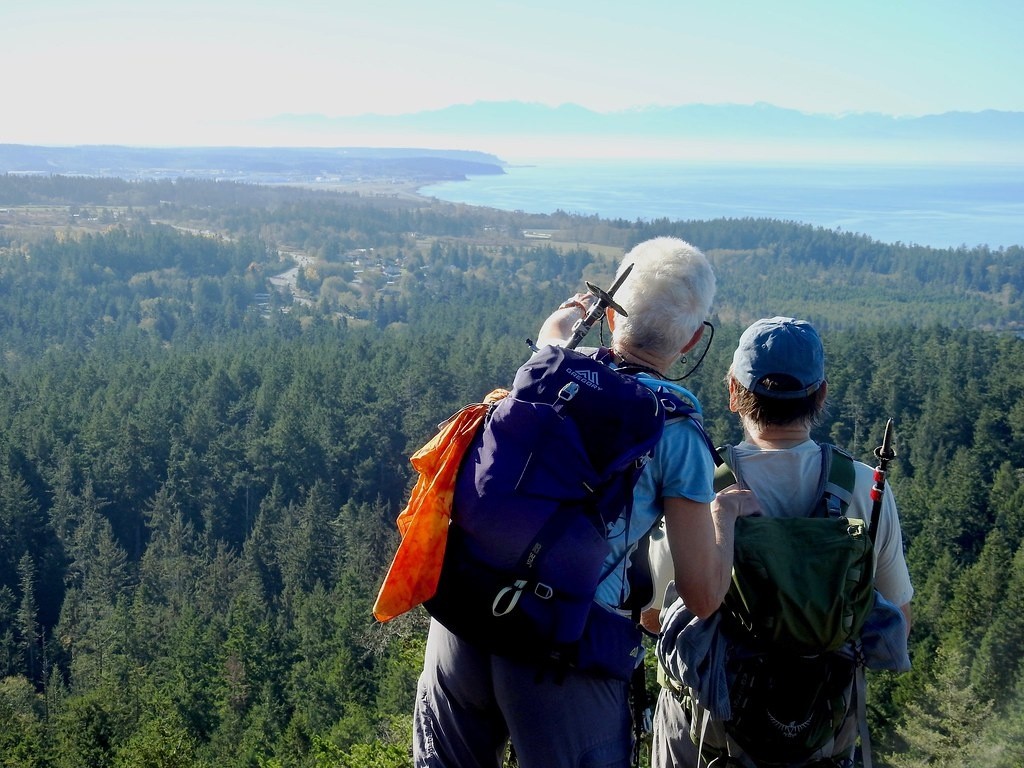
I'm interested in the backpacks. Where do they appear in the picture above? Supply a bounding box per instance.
[421,344,724,685]
[715,443,878,768]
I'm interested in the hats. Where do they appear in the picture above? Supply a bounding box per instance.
[733,316,824,399]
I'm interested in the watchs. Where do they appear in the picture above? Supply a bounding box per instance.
[558,299,586,317]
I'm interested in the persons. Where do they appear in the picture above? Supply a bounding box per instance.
[413,235,752,768]
[640,315,914,768]
